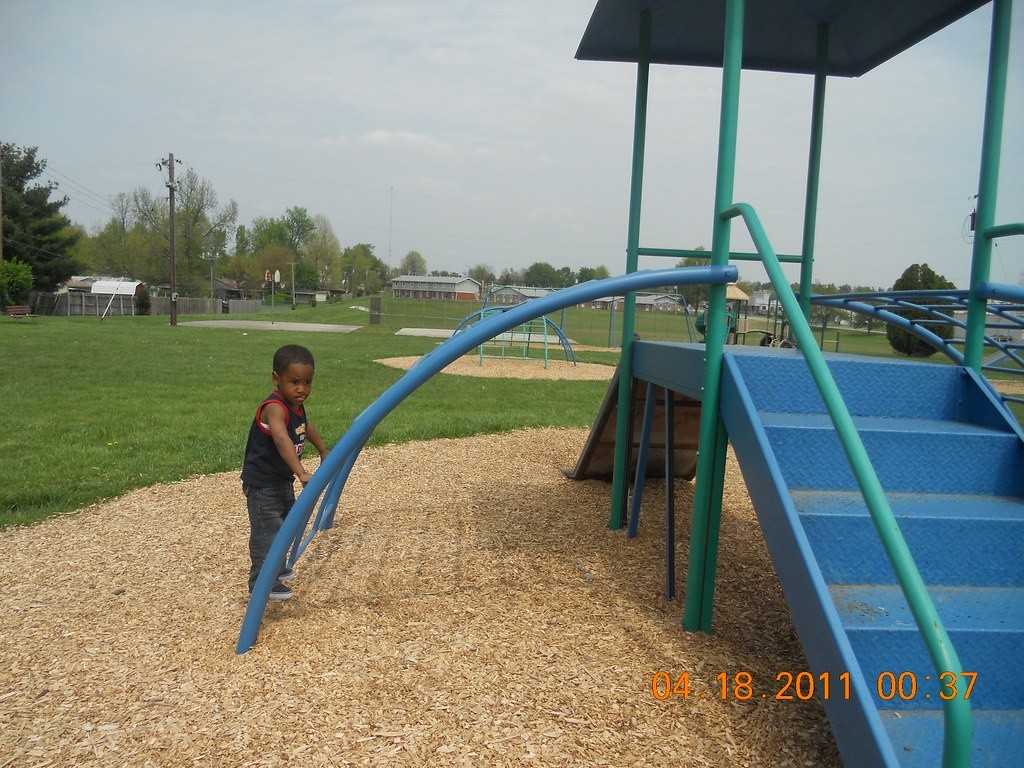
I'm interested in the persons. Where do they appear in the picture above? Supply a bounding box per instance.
[240,344,330,598]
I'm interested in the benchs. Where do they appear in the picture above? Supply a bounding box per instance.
[7,305,38,323]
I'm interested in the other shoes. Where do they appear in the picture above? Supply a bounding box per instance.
[250,578,293,599]
[278,569,295,581]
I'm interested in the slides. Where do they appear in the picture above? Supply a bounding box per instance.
[560,332,702,482]
